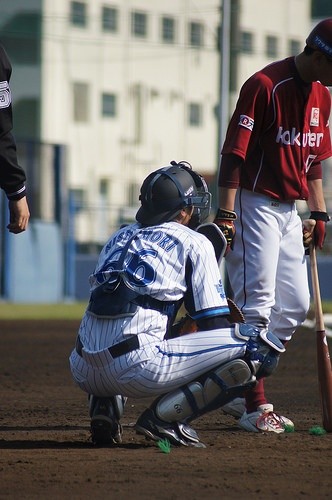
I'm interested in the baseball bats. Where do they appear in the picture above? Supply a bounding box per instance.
[303,217,332,433]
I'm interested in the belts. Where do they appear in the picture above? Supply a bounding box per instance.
[73,336,139,362]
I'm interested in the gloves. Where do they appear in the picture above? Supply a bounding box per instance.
[302,210,332,255]
[212,207,237,257]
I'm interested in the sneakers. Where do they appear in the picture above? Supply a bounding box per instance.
[87,397,126,449]
[238,403,285,435]
[133,407,191,448]
[221,396,246,419]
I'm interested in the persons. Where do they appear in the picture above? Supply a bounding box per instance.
[214,18,331,434]
[0,45,30,235]
[68,159,286,446]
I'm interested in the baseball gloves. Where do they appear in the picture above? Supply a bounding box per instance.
[180,296,246,338]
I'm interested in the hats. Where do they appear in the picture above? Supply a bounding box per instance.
[135,164,199,226]
[306,17,332,55]
